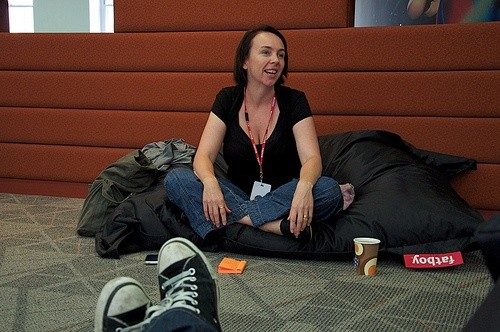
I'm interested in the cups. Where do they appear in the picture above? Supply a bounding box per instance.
[353,238,381,276]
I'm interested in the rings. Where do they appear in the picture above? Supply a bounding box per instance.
[303,214,311,218]
[219,206,226,209]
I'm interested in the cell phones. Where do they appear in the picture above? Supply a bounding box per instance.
[144,254,158,264]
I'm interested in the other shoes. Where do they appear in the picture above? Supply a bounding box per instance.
[280,217,313,242]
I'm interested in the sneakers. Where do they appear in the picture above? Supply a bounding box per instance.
[156,237,223,332]
[94,276,161,332]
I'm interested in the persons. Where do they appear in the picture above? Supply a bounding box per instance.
[93,236,224,332]
[162,24,344,243]
